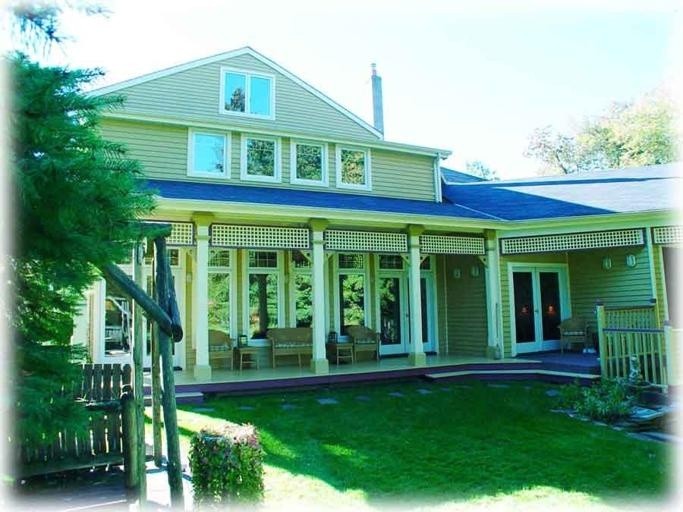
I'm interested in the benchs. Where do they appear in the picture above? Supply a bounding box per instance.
[263,328,313,369]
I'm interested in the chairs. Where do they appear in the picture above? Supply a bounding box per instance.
[344,324,380,363]
[206,330,233,372]
[556,316,592,355]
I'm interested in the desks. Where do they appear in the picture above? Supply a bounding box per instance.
[325,342,353,368]
[233,346,259,372]
